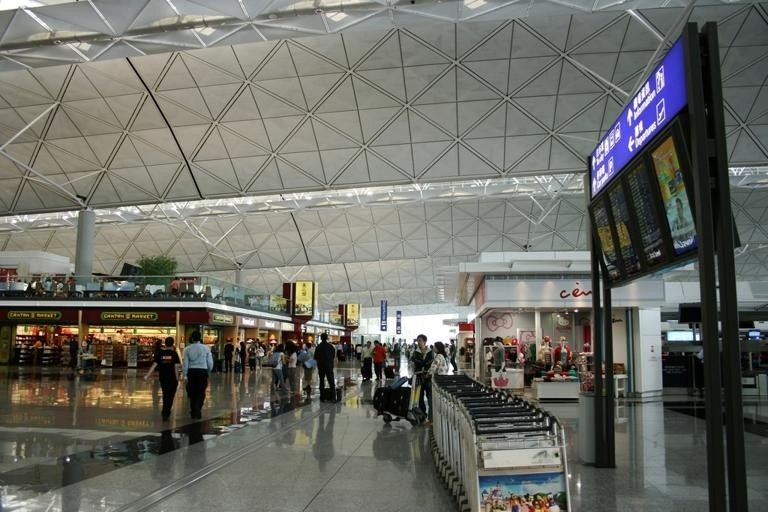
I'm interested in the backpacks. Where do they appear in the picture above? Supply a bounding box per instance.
[268,352,279,367]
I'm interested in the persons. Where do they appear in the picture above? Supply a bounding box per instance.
[32,335,43,364]
[148,374,162,414]
[9,272,75,299]
[553,339,571,365]
[425,340,449,376]
[40,336,48,347]
[200,336,458,392]
[478,478,562,512]
[143,336,184,422]
[670,197,693,243]
[153,339,165,373]
[488,336,507,372]
[409,334,437,426]
[68,335,78,368]
[539,338,556,364]
[268,394,316,449]
[181,417,209,472]
[169,276,194,299]
[182,330,214,420]
[140,422,179,489]
[311,332,337,404]
[310,400,339,472]
[209,367,411,421]
[407,420,432,507]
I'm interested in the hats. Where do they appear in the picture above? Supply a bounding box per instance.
[492,336,503,342]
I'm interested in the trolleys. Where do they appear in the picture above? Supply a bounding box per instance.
[377,367,428,426]
[428,368,577,512]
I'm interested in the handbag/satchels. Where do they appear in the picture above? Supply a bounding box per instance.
[447,360,454,376]
[494,378,509,386]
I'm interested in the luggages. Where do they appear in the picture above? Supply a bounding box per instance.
[324,389,342,401]
[384,360,395,378]
[373,387,410,416]
[360,361,372,378]
